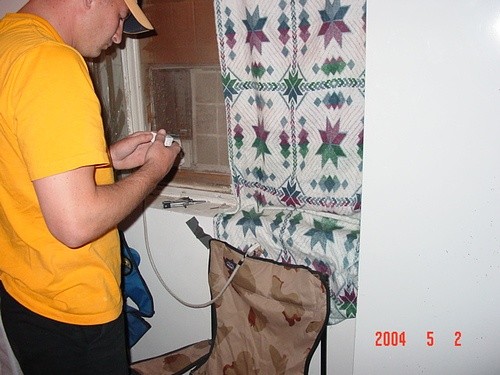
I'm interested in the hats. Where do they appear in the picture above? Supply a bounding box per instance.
[124,0,155,36]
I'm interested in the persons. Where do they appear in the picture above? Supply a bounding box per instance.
[0,0,181,375]
